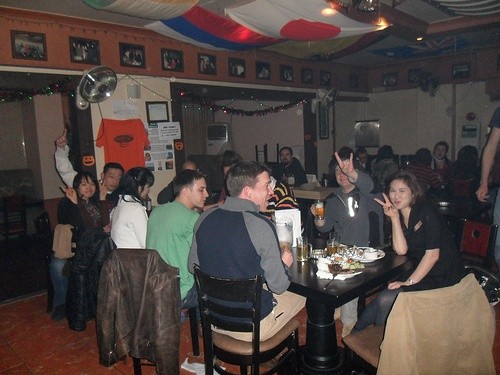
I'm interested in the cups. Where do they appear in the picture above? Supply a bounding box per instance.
[296,236,313,262]
[329,232,340,243]
[326,240,340,256]
[314,199,324,220]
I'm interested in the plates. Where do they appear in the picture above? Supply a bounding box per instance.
[346,247,387,262]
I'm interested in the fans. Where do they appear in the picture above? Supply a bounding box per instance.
[77,66,118,110]
[417,71,438,96]
[318,87,338,109]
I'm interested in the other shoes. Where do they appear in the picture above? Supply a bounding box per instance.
[342,319,357,337]
[333,306,340,321]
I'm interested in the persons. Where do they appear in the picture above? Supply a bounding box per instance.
[475,107,500,275]
[46,127,496,336]
[187,162,341,343]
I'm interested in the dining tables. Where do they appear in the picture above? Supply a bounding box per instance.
[290,186,337,212]
[281,248,416,375]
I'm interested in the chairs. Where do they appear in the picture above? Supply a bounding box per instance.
[0,196,26,249]
[36,153,500,375]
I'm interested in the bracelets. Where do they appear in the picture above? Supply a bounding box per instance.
[406,278,414,286]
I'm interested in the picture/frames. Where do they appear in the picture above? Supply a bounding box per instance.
[256,62,270,80]
[197,53,216,74]
[228,57,246,77]
[302,68,313,83]
[11,30,48,61]
[280,65,293,81]
[69,37,101,65]
[119,43,145,68]
[161,48,184,72]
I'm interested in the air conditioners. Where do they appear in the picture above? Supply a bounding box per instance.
[206,124,229,155]
